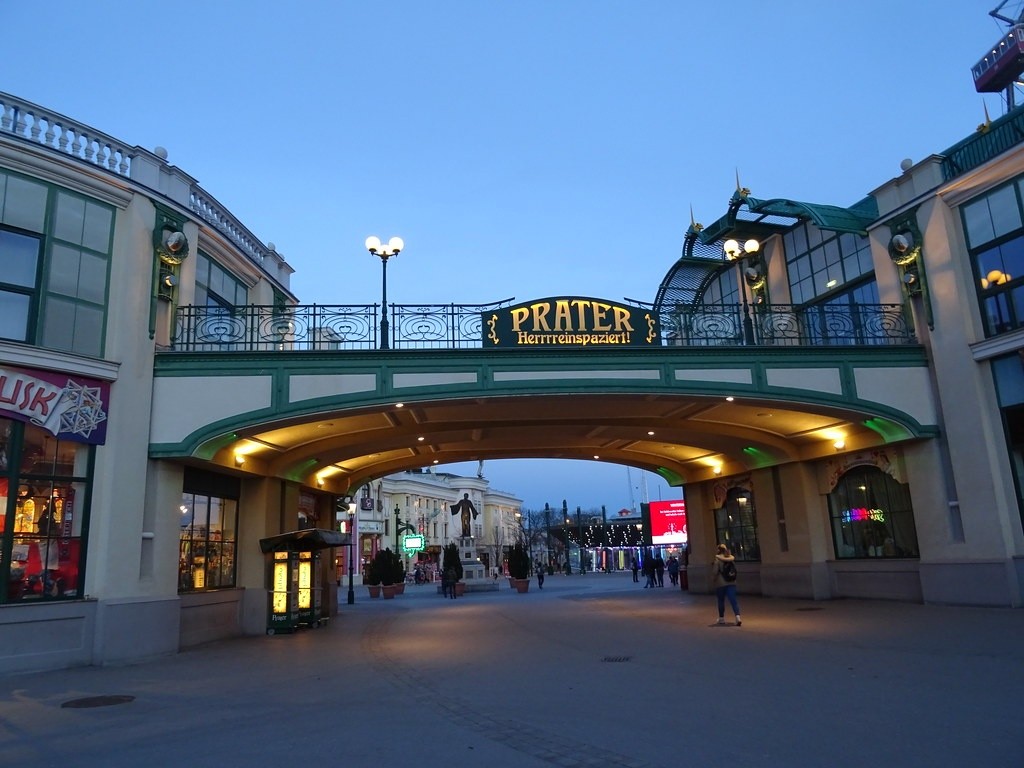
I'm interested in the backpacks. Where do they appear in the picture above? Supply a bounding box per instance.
[718,562,737,581]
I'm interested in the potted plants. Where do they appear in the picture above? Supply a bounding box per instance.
[515,544,530,593]
[375,547,396,599]
[509,545,515,589]
[394,554,407,594]
[445,542,466,597]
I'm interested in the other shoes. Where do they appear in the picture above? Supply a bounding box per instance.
[717,619,725,624]
[734,617,742,626]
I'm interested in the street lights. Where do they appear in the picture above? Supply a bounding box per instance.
[365,235,405,349]
[345,501,356,606]
[724,239,760,343]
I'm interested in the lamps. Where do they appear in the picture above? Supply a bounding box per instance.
[903,274,916,284]
[235,456,245,467]
[317,479,325,488]
[368,560,381,598]
[753,295,764,305]
[166,275,177,286]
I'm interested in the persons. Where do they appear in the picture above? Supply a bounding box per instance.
[413,563,422,584]
[629,553,679,587]
[537,562,572,589]
[447,569,458,599]
[712,544,742,626]
